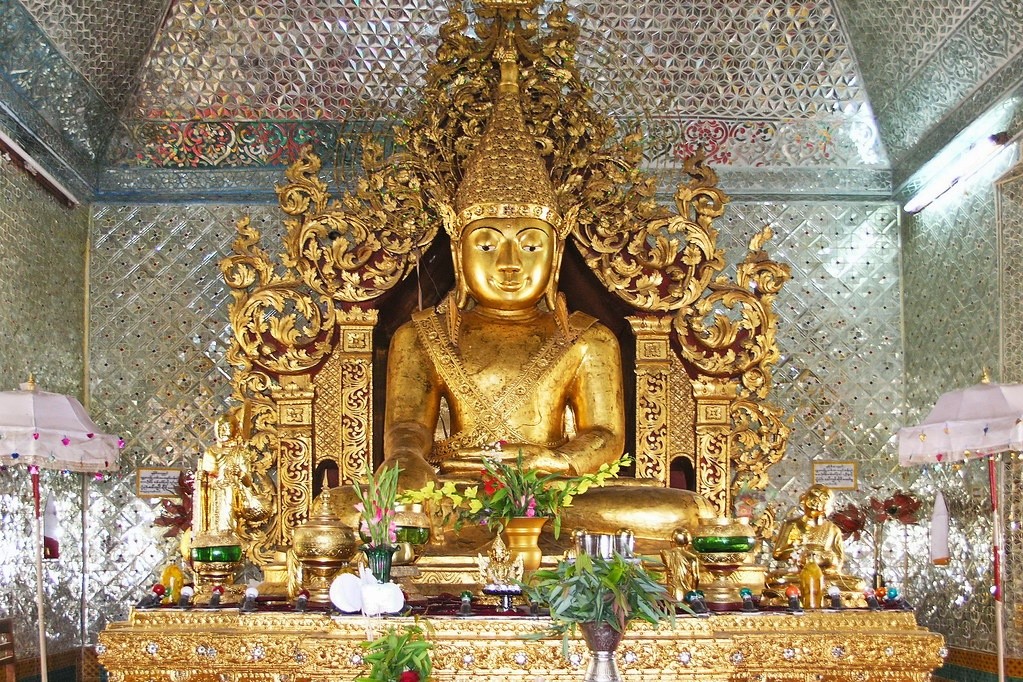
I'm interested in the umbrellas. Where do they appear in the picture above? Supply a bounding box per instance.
[0,373,121,682]
[894,363,1022,682]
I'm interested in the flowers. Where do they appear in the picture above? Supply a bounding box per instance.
[392,440,635,541]
[348,459,406,569]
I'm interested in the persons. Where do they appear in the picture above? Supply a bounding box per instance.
[189,410,264,542]
[770,486,861,594]
[313,48,719,591]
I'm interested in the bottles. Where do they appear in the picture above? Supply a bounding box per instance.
[798,553,823,609]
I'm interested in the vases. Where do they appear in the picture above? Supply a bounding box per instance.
[358,542,402,584]
[494,515,551,574]
